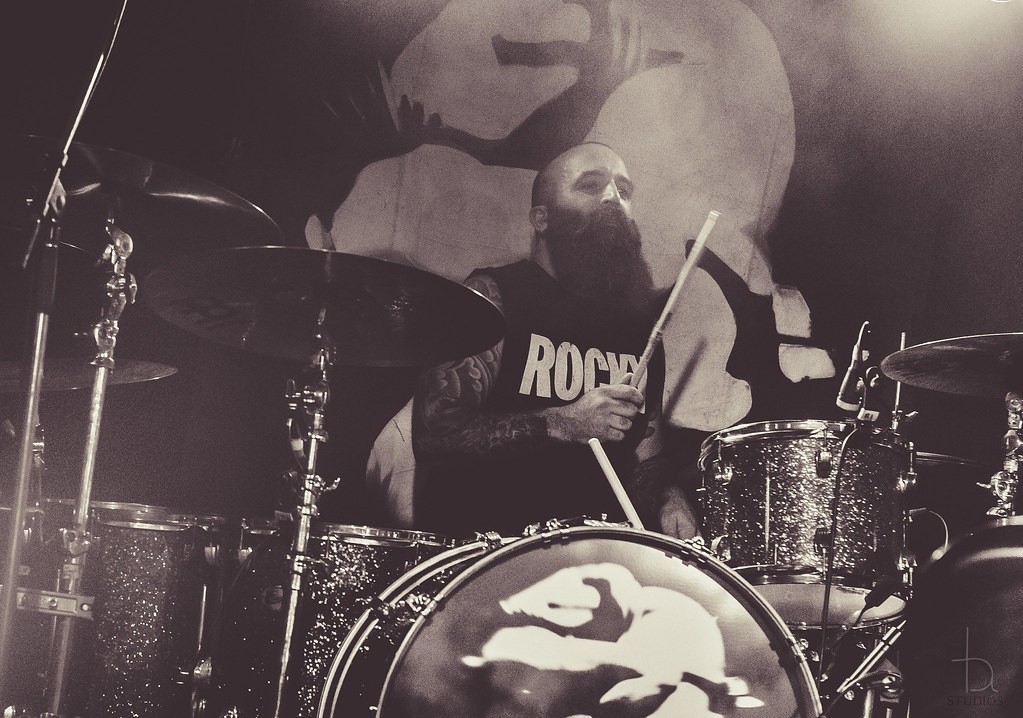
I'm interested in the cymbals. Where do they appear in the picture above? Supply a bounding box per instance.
[1,177,280,394]
[145,243,508,367]
[879,333,1022,395]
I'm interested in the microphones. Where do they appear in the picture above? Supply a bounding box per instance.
[836,321,872,412]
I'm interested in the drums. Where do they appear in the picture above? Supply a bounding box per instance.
[749,583,905,716]
[12,494,259,717]
[229,514,444,717]
[694,417,920,629]
[313,510,824,718]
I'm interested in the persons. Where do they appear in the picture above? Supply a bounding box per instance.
[412,142,704,548]
